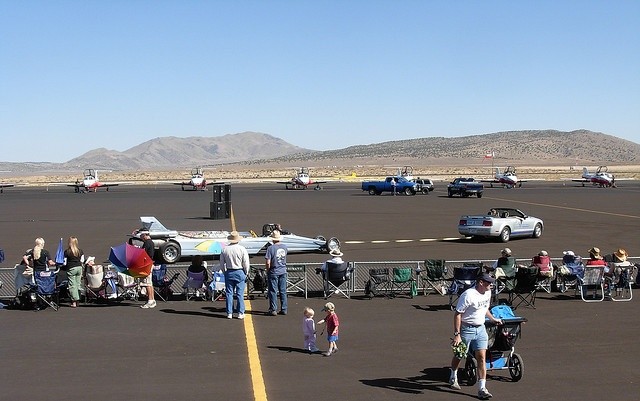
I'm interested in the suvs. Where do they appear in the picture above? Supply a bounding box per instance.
[408,179,434,194]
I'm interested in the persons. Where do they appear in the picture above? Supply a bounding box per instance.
[605,248,631,296]
[22,237,57,310]
[529,251,553,267]
[570,247,605,296]
[64,237,85,308]
[556,250,576,275]
[321,248,345,296]
[492,257,516,290]
[186,256,209,284]
[264,230,289,316]
[317,302,339,357]
[303,308,319,353]
[484,248,514,271]
[220,231,251,319]
[139,232,157,309]
[84,255,95,267]
[448,271,504,399]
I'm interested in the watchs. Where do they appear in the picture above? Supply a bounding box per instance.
[454,332,460,335]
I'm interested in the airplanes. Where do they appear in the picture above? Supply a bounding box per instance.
[66,169,118,193]
[571,166,634,188]
[277,167,326,190]
[481,166,527,188]
[172,168,224,190]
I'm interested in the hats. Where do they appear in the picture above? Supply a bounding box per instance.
[501,248,511,257]
[136,230,149,236]
[83,256,95,264]
[267,230,284,241]
[321,302,334,311]
[563,250,577,257]
[614,248,628,262]
[329,249,343,256]
[538,250,548,256]
[227,231,243,243]
[588,246,603,259]
[474,274,496,283]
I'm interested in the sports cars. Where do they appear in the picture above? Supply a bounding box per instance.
[458,207,544,243]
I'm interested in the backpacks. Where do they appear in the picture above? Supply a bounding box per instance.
[619,265,629,289]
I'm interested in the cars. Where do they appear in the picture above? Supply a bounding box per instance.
[126,217,340,264]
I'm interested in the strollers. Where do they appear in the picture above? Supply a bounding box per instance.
[464,305,528,386]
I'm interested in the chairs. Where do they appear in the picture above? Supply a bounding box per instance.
[363,267,392,300]
[286,264,308,300]
[210,271,227,303]
[181,270,209,302]
[153,272,180,303]
[13,263,35,296]
[574,265,606,303]
[57,264,69,307]
[498,256,516,267]
[84,263,107,304]
[492,267,516,306]
[529,258,553,294]
[415,258,448,297]
[247,265,269,299]
[391,268,417,299]
[509,265,540,310]
[463,262,483,271]
[321,260,352,300]
[555,264,584,294]
[604,264,637,302]
[115,272,140,303]
[447,267,481,311]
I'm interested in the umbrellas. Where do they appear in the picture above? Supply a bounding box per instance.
[56,238,65,264]
[107,242,153,278]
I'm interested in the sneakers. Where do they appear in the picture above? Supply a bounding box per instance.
[279,310,288,315]
[478,388,492,399]
[325,351,332,356]
[140,301,157,308]
[332,349,339,354]
[228,313,233,318]
[449,377,461,389]
[268,310,277,316]
[238,312,244,319]
[108,294,117,299]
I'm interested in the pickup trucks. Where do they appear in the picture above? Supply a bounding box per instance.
[447,178,484,198]
[362,176,420,196]
